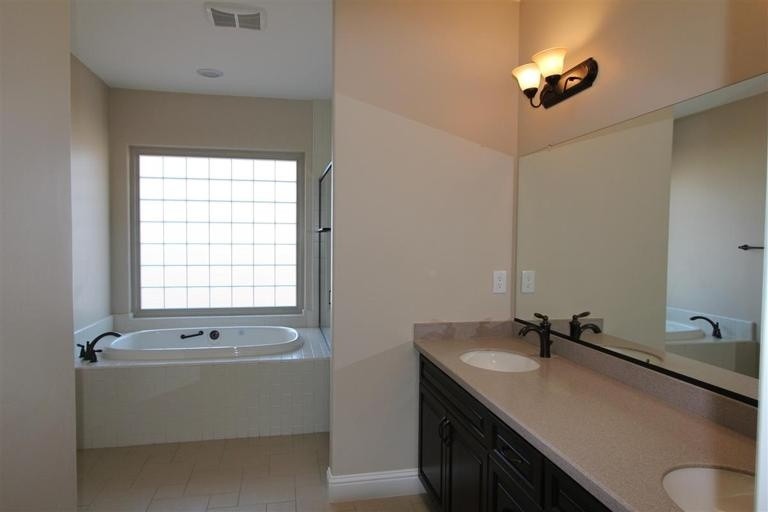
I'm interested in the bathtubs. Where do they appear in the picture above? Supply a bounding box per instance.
[667,320,705,340]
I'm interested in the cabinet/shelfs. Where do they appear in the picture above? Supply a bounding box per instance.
[417,354,489,512]
[491,415,612,512]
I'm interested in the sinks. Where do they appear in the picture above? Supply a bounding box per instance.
[459,348,541,374]
[103,325,305,360]
[604,345,662,363]
[663,463,756,512]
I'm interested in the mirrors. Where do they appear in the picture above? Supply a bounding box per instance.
[512,69,768,410]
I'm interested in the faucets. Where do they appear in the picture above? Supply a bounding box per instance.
[569,311,601,340]
[690,315,722,337]
[518,313,552,358]
[83,331,121,360]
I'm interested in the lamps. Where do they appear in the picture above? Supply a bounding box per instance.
[509,44,599,111]
